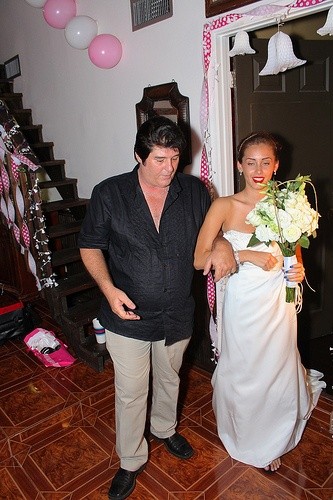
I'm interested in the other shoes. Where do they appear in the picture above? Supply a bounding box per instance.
[264,458,281,472]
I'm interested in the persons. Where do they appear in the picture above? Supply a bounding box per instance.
[77,116,234,500]
[193,130,327,471]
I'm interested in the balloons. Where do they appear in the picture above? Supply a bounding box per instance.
[43,0,76,29]
[88,34,122,69]
[25,0,48,8]
[64,15,98,51]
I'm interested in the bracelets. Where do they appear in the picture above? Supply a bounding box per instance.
[234,250,241,265]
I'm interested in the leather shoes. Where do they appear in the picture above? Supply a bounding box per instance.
[149,424,193,460]
[108,462,144,500]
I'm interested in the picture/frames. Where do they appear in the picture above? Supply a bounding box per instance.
[135,82,193,172]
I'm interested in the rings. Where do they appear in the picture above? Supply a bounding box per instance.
[298,273,301,277]
[227,273,231,277]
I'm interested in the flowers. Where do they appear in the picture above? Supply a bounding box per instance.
[244,173,322,305]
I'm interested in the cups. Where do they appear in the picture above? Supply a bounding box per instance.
[92,318,106,344]
